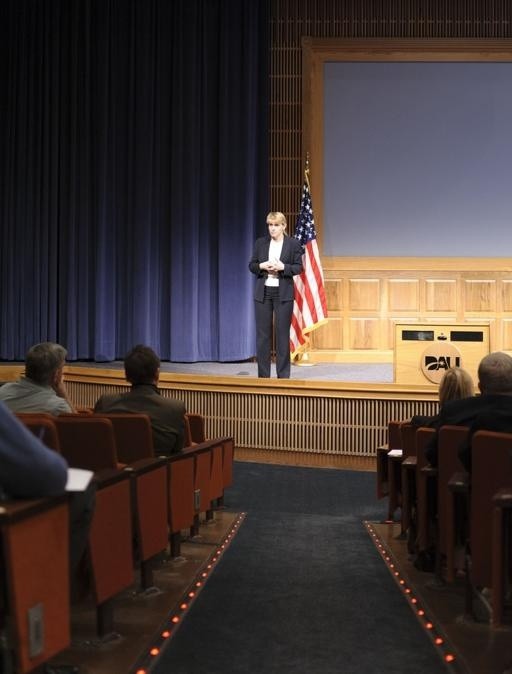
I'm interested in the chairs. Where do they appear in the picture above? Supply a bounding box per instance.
[0,399,239,674]
[367,408,511,638]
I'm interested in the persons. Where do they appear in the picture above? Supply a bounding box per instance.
[458,399,510,495]
[249,210,302,379]
[0,340,81,417]
[96,342,187,457]
[398,369,474,431]
[424,350,511,468]
[1,400,99,674]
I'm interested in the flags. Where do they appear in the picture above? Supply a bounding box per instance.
[287,168,328,362]
